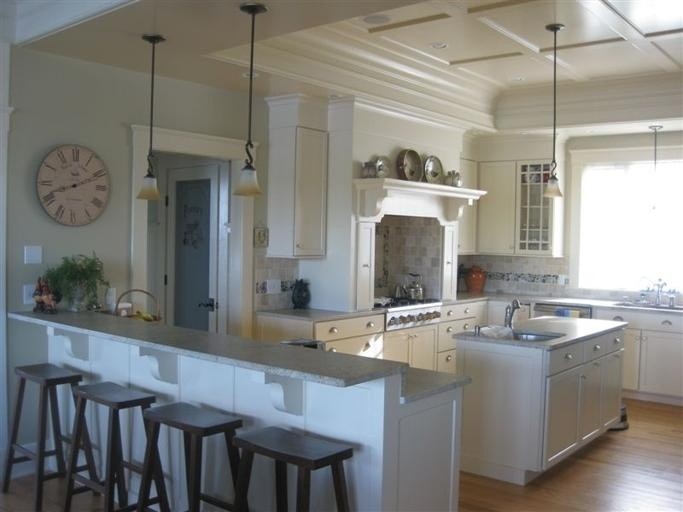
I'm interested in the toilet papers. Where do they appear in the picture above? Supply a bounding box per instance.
[105,286,118,307]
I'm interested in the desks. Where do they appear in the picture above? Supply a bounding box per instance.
[3,306,415,512]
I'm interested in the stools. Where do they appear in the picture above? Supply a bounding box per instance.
[135,401,243,510]
[0,362,101,511]
[60,381,171,512]
[232,426,352,512]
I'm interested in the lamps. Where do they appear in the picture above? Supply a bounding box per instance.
[134,32,166,201]
[538,20,563,200]
[229,3,272,193]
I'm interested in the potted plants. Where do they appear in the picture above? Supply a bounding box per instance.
[46,249,111,314]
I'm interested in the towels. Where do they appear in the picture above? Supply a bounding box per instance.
[554,306,569,317]
[563,310,580,318]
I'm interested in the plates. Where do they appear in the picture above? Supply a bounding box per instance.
[374,156,392,179]
[424,155,443,184]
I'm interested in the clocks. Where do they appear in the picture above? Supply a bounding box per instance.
[35,142,111,227]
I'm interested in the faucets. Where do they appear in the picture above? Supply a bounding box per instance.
[654,278,667,303]
[501,298,523,330]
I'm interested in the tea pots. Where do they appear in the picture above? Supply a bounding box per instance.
[402,272,425,301]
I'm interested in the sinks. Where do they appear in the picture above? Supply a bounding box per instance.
[500,327,565,344]
[613,301,683,312]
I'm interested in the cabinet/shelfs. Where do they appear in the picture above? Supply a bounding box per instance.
[473,161,517,256]
[257,312,385,361]
[382,324,437,375]
[579,335,605,452]
[594,305,682,409]
[260,93,327,262]
[603,327,621,438]
[446,340,583,487]
[455,157,479,259]
[438,299,487,373]
[512,160,563,258]
[485,300,530,328]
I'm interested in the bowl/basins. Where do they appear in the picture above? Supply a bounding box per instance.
[394,150,425,182]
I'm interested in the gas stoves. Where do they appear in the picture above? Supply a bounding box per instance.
[374,296,442,331]
